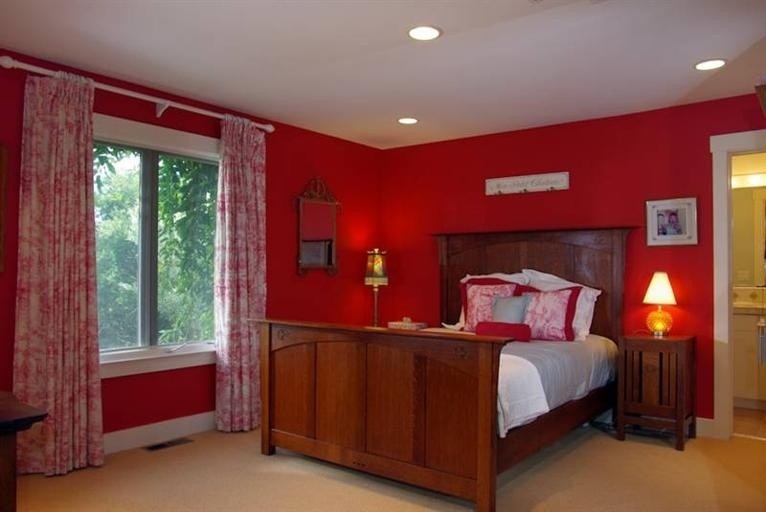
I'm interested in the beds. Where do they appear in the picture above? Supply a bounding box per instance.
[255,223,634,511]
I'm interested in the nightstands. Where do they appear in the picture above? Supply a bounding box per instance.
[615,335,698,449]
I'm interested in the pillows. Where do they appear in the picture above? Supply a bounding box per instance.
[441,267,603,343]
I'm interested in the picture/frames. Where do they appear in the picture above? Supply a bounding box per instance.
[643,197,697,247]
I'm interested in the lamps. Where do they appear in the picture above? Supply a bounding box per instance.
[640,270,677,337]
[362,248,389,326]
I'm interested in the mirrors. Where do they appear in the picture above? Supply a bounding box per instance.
[732,185,765,288]
[295,177,341,275]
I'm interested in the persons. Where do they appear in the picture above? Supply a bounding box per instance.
[657,212,683,235]
